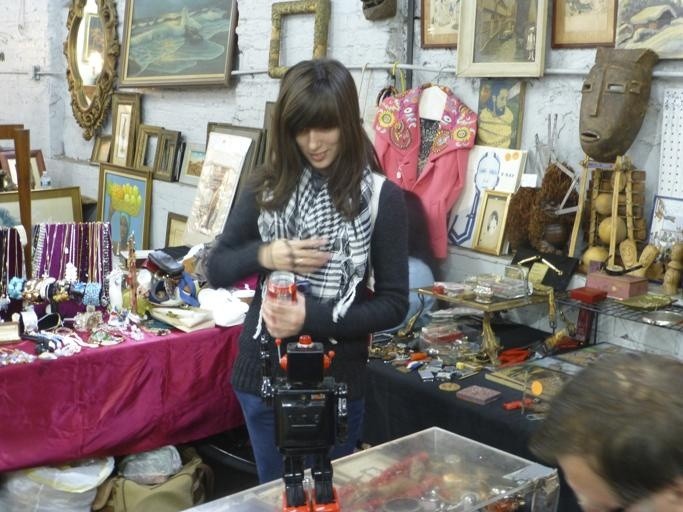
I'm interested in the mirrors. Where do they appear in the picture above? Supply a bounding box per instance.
[63,1,121,141]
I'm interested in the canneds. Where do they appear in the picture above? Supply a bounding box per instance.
[265,269,298,304]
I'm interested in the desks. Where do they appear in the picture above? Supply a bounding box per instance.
[361,318,594,512]
[177,426,560,512]
[0,272,259,471]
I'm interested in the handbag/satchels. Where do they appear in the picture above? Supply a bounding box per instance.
[92,445,214,512]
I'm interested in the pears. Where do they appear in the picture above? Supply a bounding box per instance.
[122,183,141,206]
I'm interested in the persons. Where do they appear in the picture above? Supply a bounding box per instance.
[488,211,497,234]
[476,81,514,148]
[107,194,130,256]
[377,190,438,333]
[206,60,410,484]
[528,352,683,512]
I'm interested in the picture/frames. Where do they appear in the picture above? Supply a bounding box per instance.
[87,93,207,187]
[95,161,154,255]
[2,185,84,233]
[1,124,51,191]
[473,190,513,257]
[116,1,239,88]
[180,121,267,249]
[164,210,190,252]
[419,0,683,78]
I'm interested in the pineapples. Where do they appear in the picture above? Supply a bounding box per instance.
[104,180,124,200]
